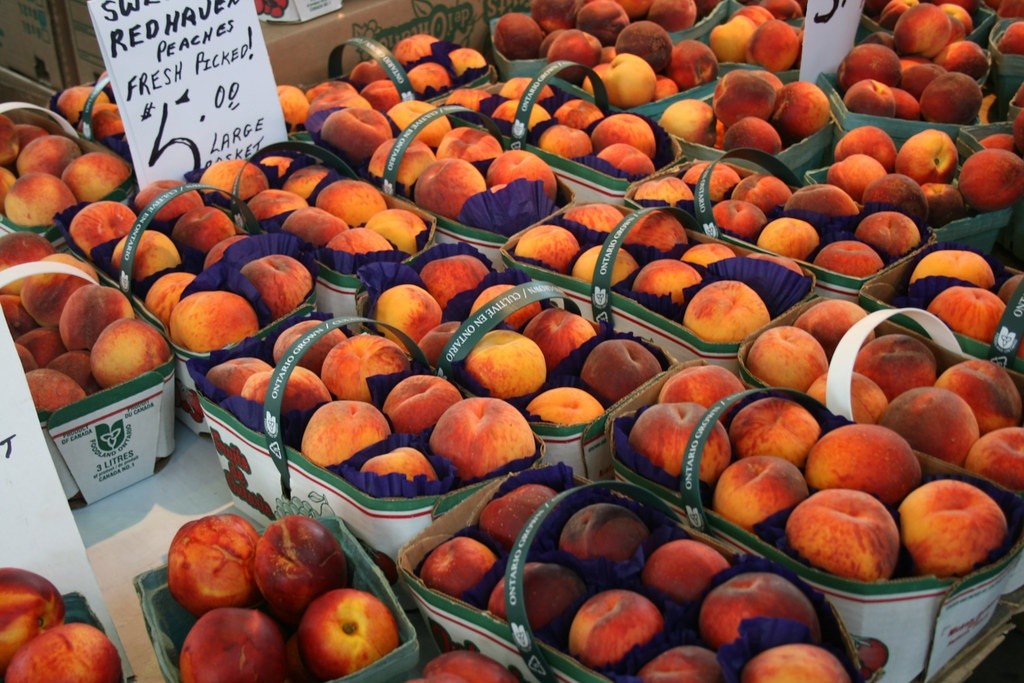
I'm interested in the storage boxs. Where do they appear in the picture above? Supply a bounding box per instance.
[0,0,533,118]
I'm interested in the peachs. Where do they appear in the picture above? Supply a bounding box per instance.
[0,0,1023,683]
